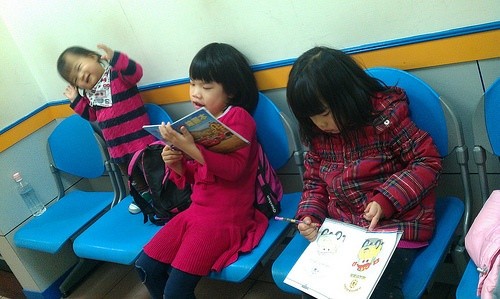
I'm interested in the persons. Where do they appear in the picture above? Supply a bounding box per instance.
[136,43,270,299]
[286,46,444,299]
[57,44,157,215]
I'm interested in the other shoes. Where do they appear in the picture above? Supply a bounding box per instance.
[128,190,152,214]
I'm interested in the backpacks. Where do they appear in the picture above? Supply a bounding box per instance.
[253,140,283,217]
[127,140,193,226]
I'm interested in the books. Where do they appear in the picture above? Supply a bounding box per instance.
[283,218,404,299]
[142,105,250,161]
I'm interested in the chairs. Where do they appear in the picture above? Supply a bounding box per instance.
[14,67,500,299]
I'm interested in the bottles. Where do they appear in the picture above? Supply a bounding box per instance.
[13,173,46,216]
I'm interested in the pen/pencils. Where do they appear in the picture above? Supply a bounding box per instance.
[275,217,322,227]
